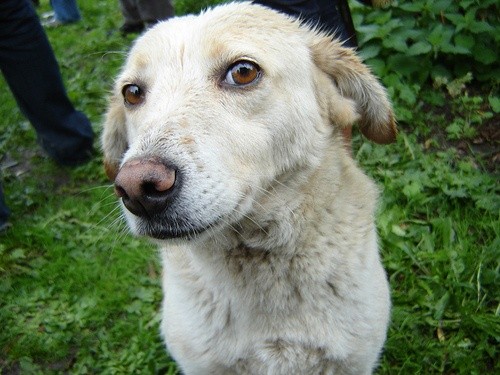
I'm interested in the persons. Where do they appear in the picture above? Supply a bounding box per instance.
[0,0,116,238]
[107,0,174,38]
[236,0,359,156]
[39,0,80,29]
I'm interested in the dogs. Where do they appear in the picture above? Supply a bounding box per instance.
[82,1,399,375]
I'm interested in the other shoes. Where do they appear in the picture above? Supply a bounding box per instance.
[42,12,54,20]
[42,18,61,28]
